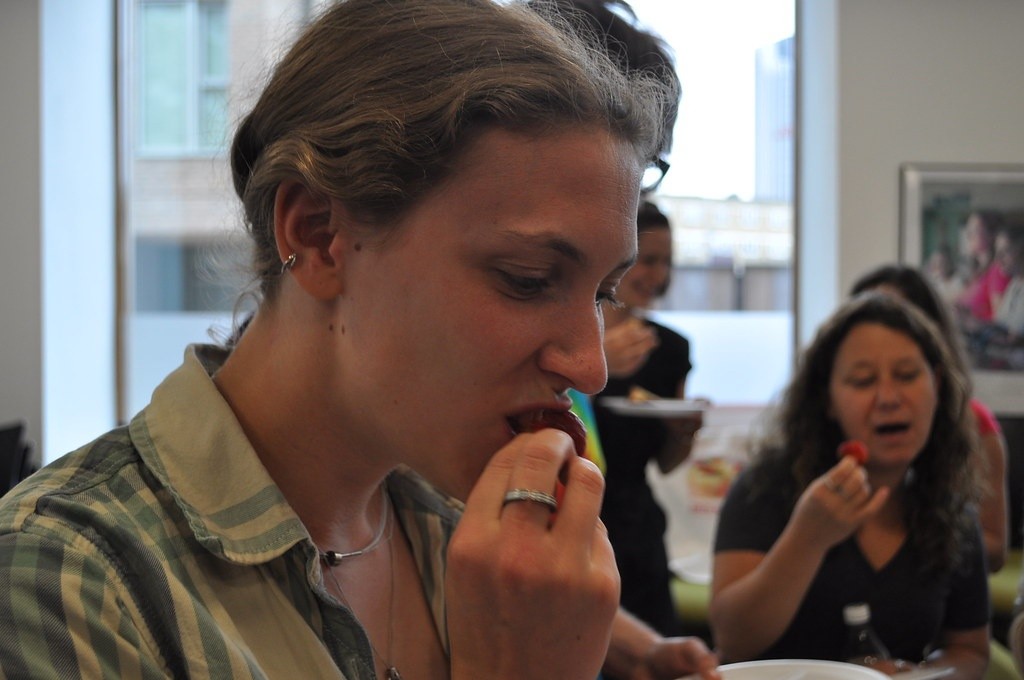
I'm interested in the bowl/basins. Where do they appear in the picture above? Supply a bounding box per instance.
[676,658,893,680]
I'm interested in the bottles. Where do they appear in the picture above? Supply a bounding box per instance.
[843,603,892,669]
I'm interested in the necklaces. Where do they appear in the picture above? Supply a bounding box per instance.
[329,493,404,680]
[318,482,388,566]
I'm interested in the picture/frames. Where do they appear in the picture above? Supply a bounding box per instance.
[896,160,1024,420]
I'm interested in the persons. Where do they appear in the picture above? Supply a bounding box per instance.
[0,0,662,680]
[574,202,715,679]
[705,289,992,680]
[850,267,1006,574]
[525,1,722,679]
[927,207,1024,370]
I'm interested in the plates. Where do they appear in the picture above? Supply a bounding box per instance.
[601,396,709,418]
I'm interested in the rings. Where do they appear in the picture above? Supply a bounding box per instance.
[503,489,559,512]
[834,485,842,494]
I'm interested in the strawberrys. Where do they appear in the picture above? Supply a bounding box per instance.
[516,408,586,455]
[837,439,868,464]
